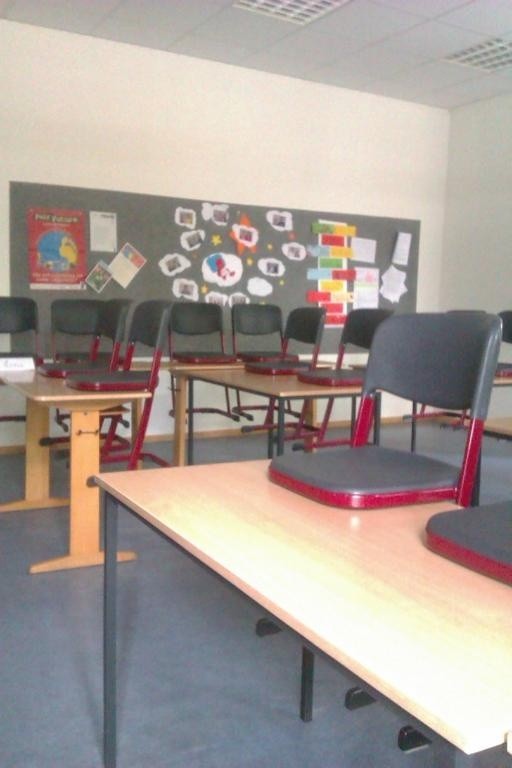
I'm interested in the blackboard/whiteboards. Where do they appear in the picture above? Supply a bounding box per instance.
[9,181,420,360]
